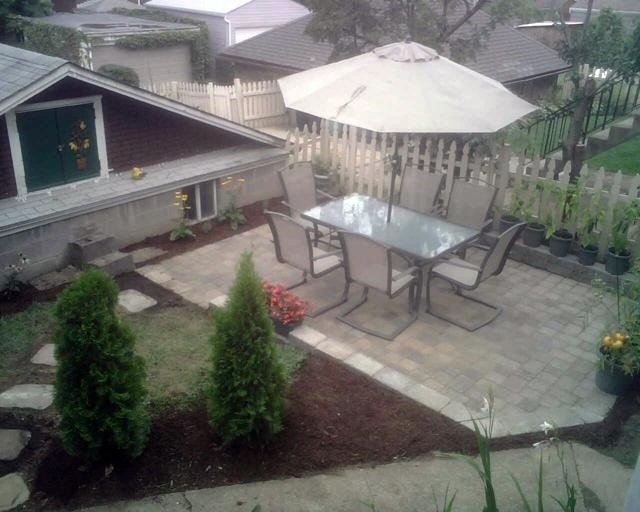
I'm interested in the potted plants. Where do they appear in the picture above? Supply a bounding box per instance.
[487,171,639,276]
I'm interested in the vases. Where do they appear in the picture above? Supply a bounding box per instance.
[595,345,640,397]
[68,118,92,158]
[77,156,88,169]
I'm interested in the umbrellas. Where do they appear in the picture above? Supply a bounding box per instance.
[275,37,542,223]
